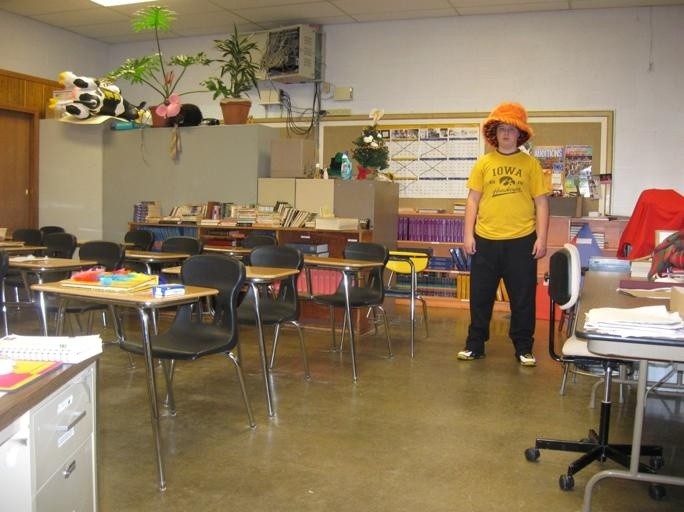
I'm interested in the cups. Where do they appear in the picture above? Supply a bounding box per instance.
[359,218,369,230]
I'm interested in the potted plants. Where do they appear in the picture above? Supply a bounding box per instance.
[203,18,265,124]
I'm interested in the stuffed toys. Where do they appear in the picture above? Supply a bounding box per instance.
[51,71,152,126]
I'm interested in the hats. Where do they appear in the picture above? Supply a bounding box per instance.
[482,102,533,148]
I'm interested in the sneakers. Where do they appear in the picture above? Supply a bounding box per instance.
[519,353,537,366]
[457,349,485,360]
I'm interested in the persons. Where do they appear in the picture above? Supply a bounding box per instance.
[457,103,549,368]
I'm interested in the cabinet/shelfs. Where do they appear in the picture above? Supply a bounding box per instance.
[382,208,519,316]
[251,175,301,209]
[292,174,375,233]
[36,118,103,243]
[103,125,276,247]
[534,211,570,323]
[569,216,635,249]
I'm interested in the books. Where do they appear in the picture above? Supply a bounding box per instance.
[570,222,606,250]
[62,201,358,295]
[395,200,507,301]
[629,260,653,278]
[0,333,103,395]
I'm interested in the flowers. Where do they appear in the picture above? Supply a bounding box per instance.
[92,8,214,122]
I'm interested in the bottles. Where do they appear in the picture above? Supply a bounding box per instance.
[313,163,321,179]
[340,152,353,180]
[323,168,328,179]
[211,205,219,220]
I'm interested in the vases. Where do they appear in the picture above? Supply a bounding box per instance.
[147,106,172,127]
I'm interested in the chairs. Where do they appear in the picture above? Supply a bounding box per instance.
[0,222,436,499]
[524,239,668,500]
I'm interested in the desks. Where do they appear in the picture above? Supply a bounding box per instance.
[573,263,684,511]
[0,334,101,510]
